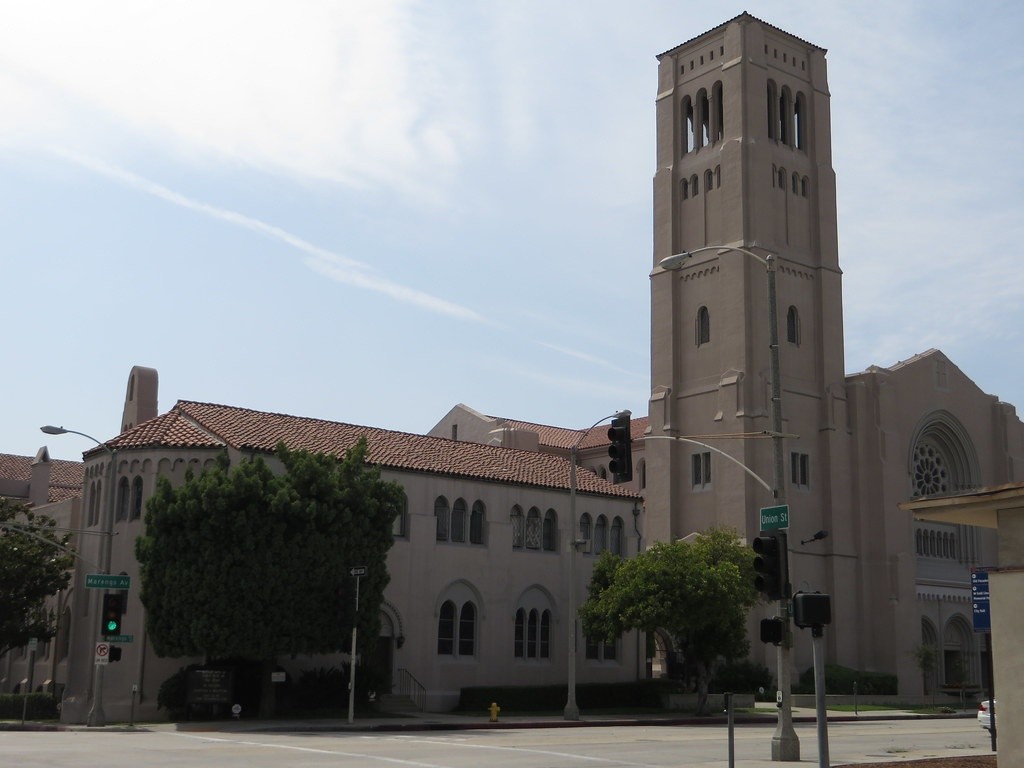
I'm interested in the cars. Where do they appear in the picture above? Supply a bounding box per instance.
[976,696,996,735]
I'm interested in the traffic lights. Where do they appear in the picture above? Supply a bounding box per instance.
[100,593,123,637]
[108,645,123,663]
[752,530,790,601]
[608,416,633,485]
[759,618,783,644]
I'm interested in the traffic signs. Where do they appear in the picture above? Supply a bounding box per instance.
[348,567,366,576]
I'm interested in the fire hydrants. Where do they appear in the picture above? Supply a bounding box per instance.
[487,703,501,723]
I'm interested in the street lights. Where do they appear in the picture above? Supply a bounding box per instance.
[40,424,119,728]
[563,408,632,720]
